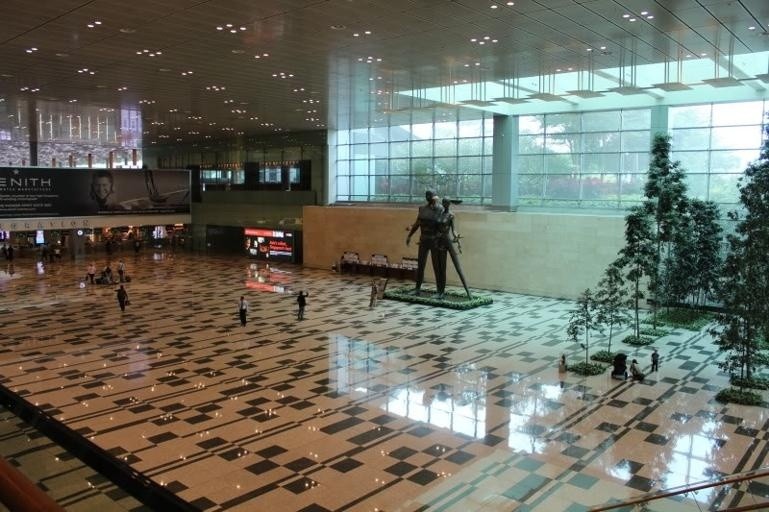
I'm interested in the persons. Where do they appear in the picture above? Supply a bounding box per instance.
[436,199,473,301]
[630,359,644,380]
[405,190,444,299]
[369,281,378,307]
[296,291,309,319]
[1,237,141,312]
[87,171,117,210]
[652,350,659,371]
[238,296,248,327]
